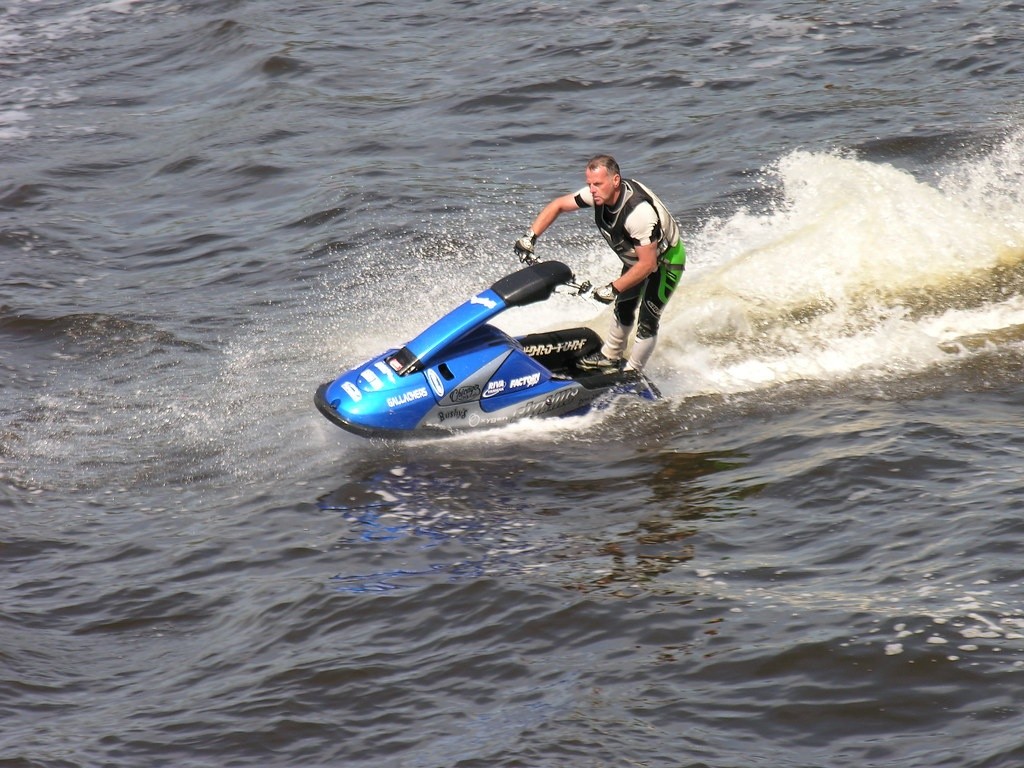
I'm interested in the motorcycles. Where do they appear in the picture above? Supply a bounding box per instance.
[312,244,664,441]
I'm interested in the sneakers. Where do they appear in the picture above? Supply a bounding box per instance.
[576,349,622,371]
[600,367,619,374]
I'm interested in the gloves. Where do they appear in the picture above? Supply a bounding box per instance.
[514,229,539,263]
[594,282,620,305]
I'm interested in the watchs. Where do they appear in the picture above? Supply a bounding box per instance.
[524,228,538,239]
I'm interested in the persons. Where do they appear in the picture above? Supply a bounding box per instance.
[513,153,687,373]
